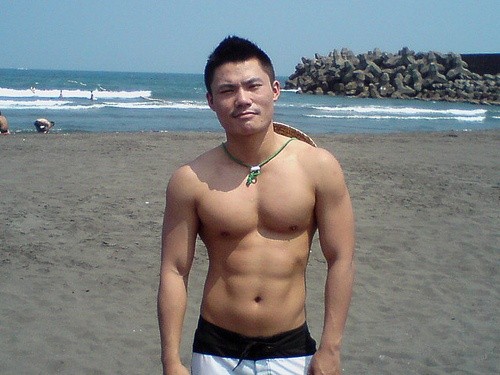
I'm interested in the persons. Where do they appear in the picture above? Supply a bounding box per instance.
[90,91,93,101]
[0,112,8,133]
[157,35,356,375]
[33,119,54,133]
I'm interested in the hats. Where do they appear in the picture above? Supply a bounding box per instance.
[273,122,318,148]
